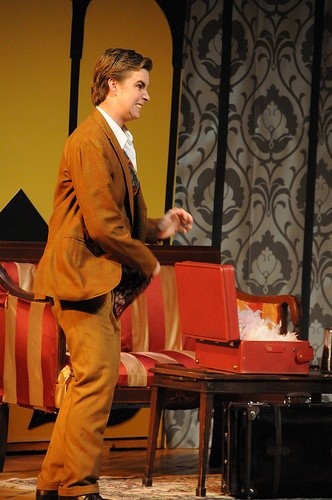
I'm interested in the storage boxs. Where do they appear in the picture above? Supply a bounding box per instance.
[175,260,314,375]
[220,396,332,499]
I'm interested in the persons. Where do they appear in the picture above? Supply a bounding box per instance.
[31,46,194,500]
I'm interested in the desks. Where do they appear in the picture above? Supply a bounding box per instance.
[142,363,332,498]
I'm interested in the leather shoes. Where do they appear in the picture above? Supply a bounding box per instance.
[34,489,115,500]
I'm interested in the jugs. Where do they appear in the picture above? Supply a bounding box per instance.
[321,327,332,374]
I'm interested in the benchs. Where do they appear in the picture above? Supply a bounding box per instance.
[0,241,303,476]
